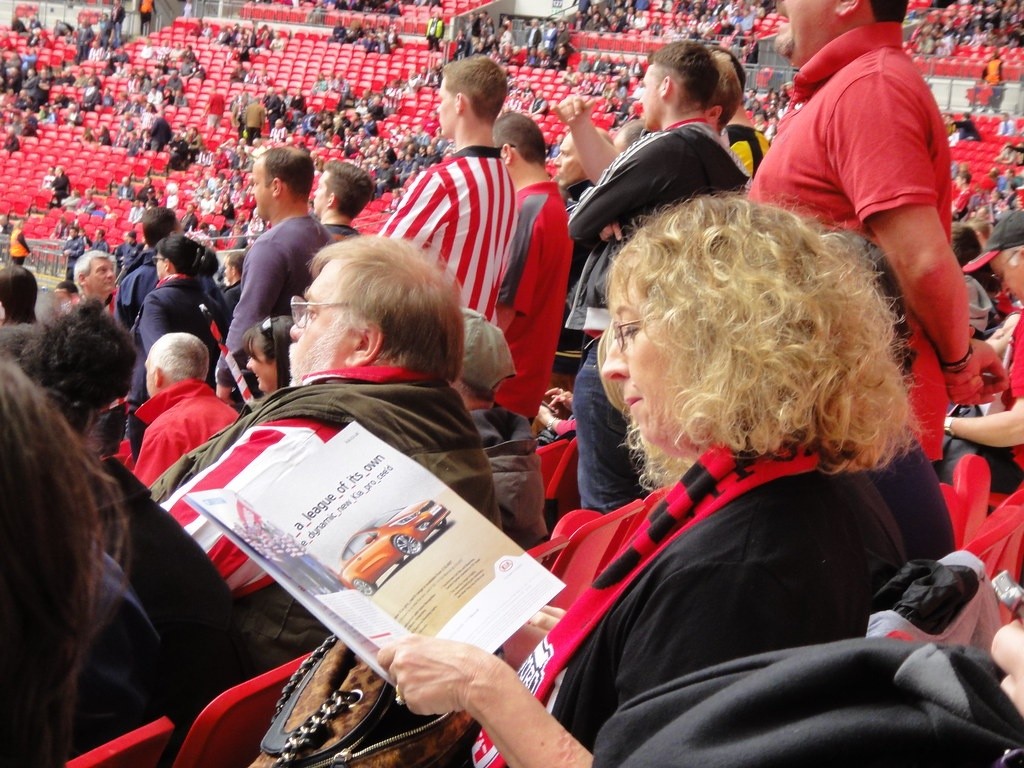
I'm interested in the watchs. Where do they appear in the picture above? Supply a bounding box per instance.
[944,417,955,436]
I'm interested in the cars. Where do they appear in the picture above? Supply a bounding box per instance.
[337,497,452,597]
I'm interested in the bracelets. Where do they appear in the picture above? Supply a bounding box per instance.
[945,342,974,373]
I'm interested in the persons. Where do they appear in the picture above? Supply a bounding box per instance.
[447,305,549,551]
[213,146,334,408]
[135,333,238,488]
[0,356,235,768]
[146,233,502,671]
[377,57,519,321]
[0,0,792,288]
[747,0,1011,461]
[242,315,292,390]
[378,195,918,768]
[492,111,574,425]
[937,210,1024,495]
[0,208,247,461]
[312,159,377,242]
[553,40,770,516]
[912,0,1024,342]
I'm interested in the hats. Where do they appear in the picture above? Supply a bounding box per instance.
[459,307,517,391]
[961,209,1024,272]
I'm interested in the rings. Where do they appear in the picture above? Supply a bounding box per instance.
[395,685,405,705]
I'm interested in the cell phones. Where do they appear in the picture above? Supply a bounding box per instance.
[992,570,1024,624]
[542,401,558,416]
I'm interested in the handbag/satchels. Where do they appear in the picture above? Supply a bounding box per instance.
[249,633,503,768]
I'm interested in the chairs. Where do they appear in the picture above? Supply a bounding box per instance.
[0,0,1024,768]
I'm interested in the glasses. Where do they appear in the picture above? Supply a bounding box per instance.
[610,316,644,353]
[259,316,274,347]
[291,295,353,329]
[151,255,165,266]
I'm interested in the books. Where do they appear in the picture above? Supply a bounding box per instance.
[181,419,566,687]
